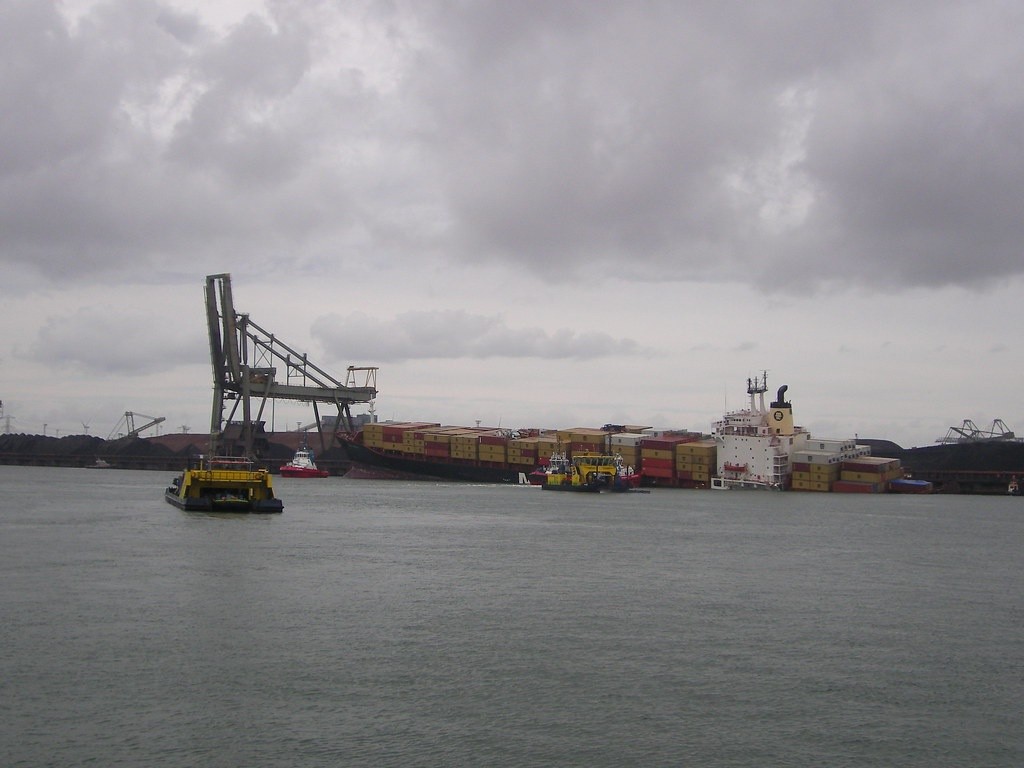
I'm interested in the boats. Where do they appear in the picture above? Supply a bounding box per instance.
[524,450,643,491]
[165,452,284,513]
[280,447,328,478]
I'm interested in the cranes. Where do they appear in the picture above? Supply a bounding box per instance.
[105,410,167,441]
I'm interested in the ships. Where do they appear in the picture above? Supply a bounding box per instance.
[335,371,930,494]
